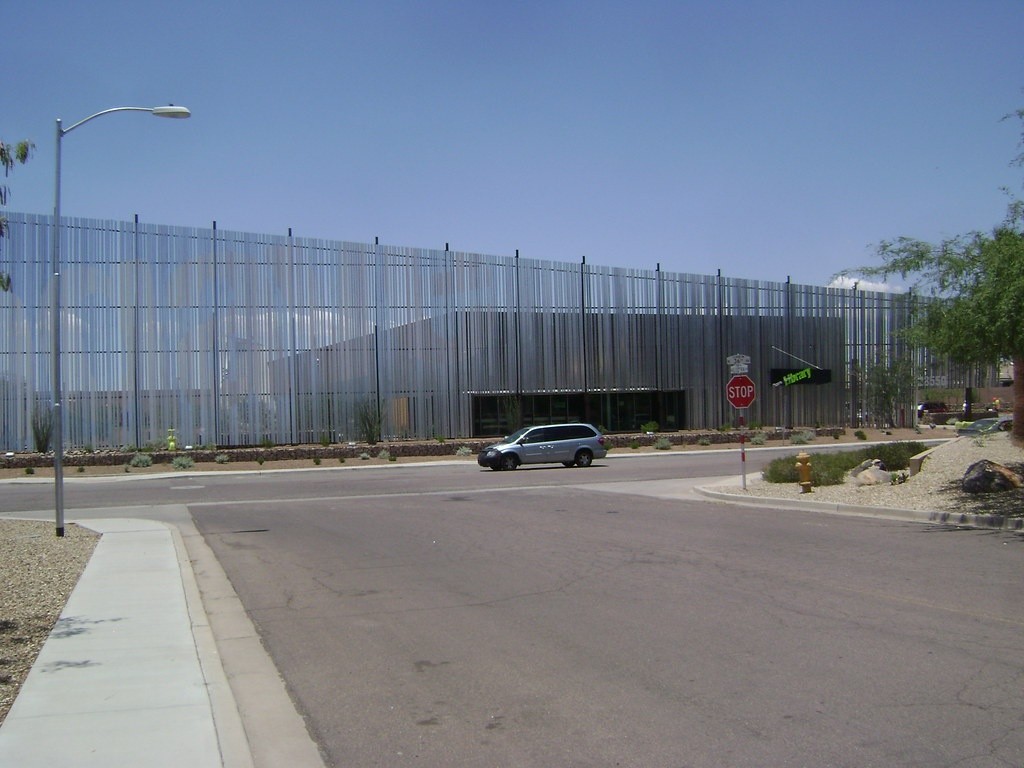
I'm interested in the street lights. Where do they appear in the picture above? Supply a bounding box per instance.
[52,106,193,538]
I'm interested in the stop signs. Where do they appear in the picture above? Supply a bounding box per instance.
[726,375,756,409]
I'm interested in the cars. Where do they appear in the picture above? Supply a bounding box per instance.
[917,401,947,416]
[478,423,608,471]
